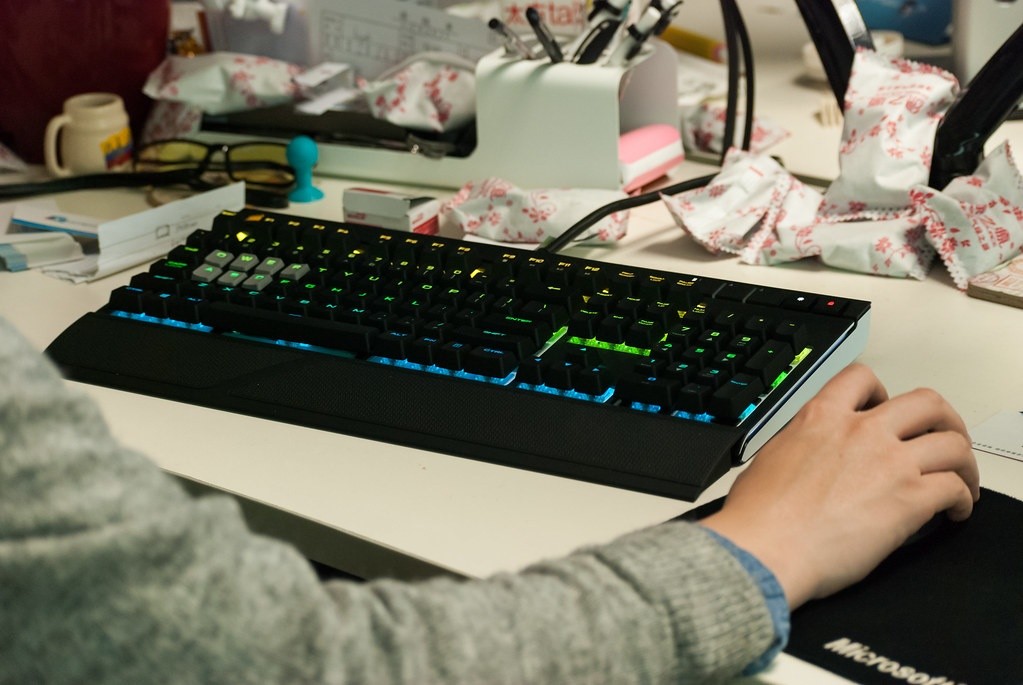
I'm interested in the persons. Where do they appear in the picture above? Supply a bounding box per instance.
[0,318,979,685]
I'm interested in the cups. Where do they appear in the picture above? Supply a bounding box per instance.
[43,93,132,176]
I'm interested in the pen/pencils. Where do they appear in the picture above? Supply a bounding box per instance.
[526,7,564,63]
[606,0,684,66]
[571,0,632,64]
[488,18,537,60]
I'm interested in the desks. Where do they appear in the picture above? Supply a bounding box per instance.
[0,1,1023,685]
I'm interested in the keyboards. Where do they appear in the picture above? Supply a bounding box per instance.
[43,205,872,500]
